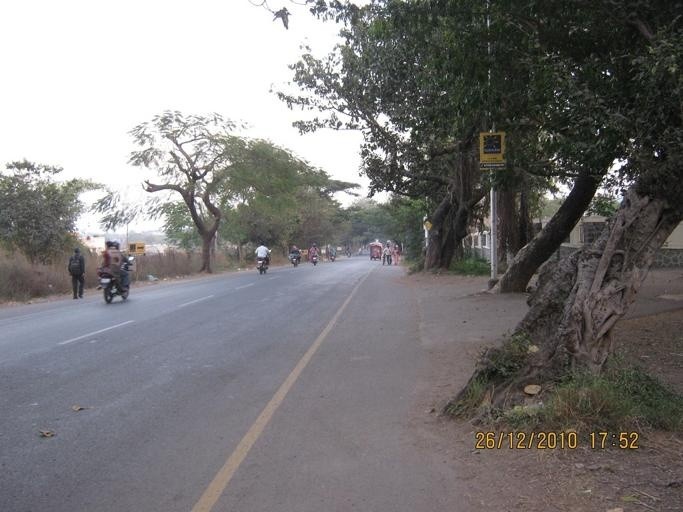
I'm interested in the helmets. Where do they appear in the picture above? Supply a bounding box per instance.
[107,241,119,250]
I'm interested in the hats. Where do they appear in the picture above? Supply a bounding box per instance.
[75,248,81,253]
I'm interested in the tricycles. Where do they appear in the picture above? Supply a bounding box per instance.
[368,242,382,260]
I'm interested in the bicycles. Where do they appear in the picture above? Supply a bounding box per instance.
[382,253,386,265]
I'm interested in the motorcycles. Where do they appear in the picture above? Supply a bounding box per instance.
[257,249,273,275]
[96,256,135,303]
[290,248,352,268]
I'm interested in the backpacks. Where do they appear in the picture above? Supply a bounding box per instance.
[70,255,81,275]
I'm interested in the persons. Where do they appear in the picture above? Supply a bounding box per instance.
[67,246,85,300]
[391,244,401,266]
[289,244,301,263]
[94,241,123,290]
[254,239,271,269]
[309,242,319,263]
[112,241,133,292]
[383,242,392,265]
[328,245,351,261]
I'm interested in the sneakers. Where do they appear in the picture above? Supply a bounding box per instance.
[73,295,83,299]
